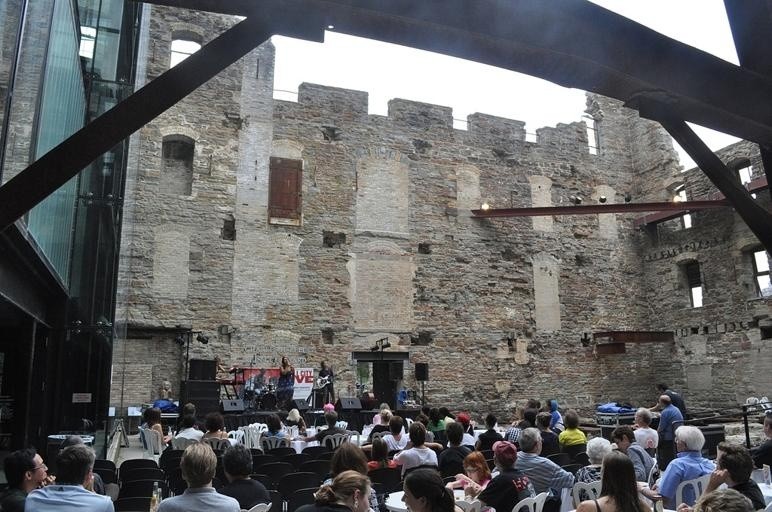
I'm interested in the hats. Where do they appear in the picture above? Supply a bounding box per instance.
[455,412,471,425]
[183,403,196,418]
[323,403,334,413]
[492,440,517,461]
[286,409,301,422]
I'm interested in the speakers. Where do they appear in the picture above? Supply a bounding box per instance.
[287,399,309,413]
[334,397,362,410]
[220,400,247,414]
[414,363,429,381]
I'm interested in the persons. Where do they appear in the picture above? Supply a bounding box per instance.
[318,359,336,408]
[254,368,268,395]
[259,402,575,512]
[523,383,771,512]
[140,382,272,512]
[1,436,115,512]
[277,357,295,412]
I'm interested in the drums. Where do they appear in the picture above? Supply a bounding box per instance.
[256,389,277,410]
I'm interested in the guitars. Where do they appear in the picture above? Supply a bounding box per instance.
[316,368,349,388]
